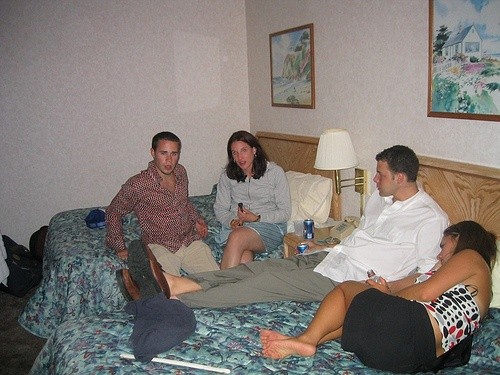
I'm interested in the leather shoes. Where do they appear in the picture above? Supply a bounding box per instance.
[127,240,170,299]
[117,269,140,302]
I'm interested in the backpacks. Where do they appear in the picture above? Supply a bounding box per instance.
[3,234,41,297]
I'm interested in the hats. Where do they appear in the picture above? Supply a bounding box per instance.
[85,208,108,227]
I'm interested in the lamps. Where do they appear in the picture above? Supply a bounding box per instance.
[313,130,366,197]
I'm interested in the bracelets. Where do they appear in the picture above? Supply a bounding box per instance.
[255,215,261,221]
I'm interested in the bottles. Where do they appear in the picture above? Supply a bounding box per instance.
[367,270,392,293]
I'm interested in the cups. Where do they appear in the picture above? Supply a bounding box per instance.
[294,219,304,236]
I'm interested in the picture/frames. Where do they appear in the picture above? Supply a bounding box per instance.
[427,0,500,122]
[269,22,314,109]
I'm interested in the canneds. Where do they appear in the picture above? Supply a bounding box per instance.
[303,219,314,239]
[297,244,309,254]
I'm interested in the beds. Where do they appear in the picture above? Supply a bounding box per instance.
[18,132,341,339]
[27,155,500,375]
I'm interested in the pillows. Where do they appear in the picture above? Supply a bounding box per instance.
[284,170,332,232]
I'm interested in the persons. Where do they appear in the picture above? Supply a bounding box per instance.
[105,132,221,276]
[259,221,496,373]
[117,145,449,308]
[213,131,292,269]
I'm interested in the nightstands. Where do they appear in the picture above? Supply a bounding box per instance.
[283,225,340,259]
